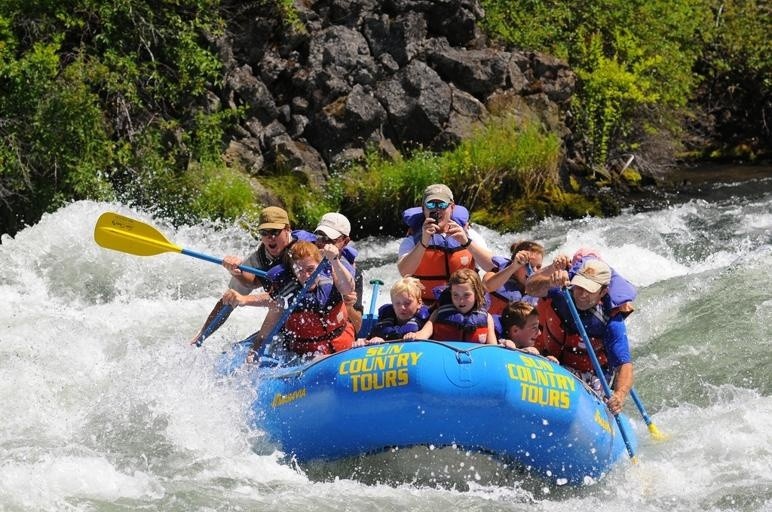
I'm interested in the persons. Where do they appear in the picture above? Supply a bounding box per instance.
[483,240,545,315]
[492,300,561,365]
[221,212,364,317]
[351,273,430,349]
[189,205,317,345]
[403,266,498,346]
[246,238,357,363]
[396,183,497,309]
[524,253,639,415]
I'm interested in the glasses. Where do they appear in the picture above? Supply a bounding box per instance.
[423,201,451,210]
[258,229,286,236]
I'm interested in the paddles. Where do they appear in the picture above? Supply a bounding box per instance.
[95,212,267,278]
[629,388,667,444]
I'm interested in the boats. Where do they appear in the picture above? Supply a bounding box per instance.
[211,316,643,504]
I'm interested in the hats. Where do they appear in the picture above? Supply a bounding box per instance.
[312,211,352,241]
[421,183,454,205]
[256,205,290,233]
[569,258,612,294]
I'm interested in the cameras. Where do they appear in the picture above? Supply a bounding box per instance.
[429,211,438,225]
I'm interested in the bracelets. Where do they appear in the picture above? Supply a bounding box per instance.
[419,238,428,249]
[460,238,472,247]
